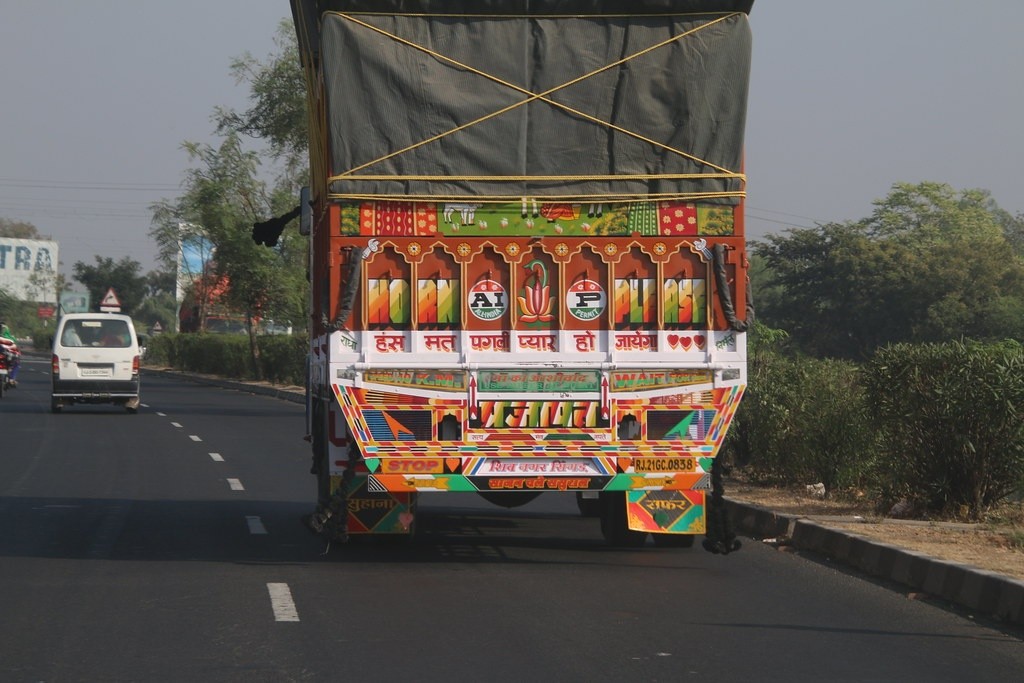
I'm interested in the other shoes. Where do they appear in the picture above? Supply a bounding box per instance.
[10,379,18,385]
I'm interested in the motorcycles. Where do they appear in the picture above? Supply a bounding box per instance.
[0,336,21,398]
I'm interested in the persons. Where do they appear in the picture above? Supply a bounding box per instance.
[0,319,21,385]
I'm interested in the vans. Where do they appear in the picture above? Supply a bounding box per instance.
[51,310,146,414]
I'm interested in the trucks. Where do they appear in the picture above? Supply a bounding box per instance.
[245,0,762,557]
